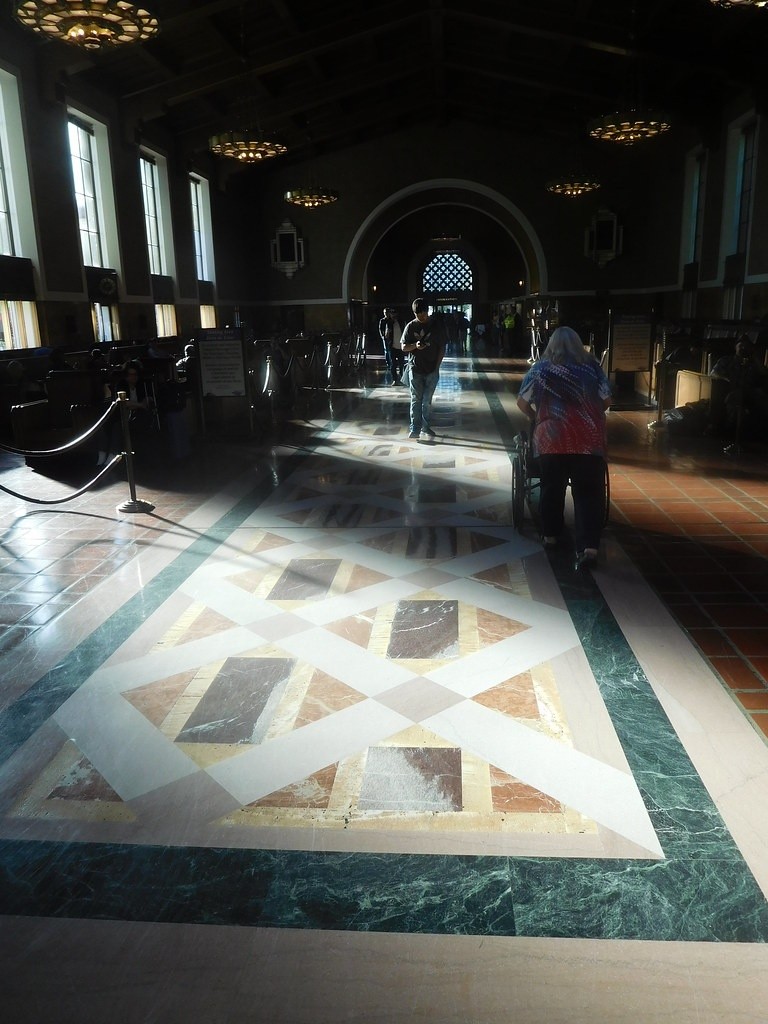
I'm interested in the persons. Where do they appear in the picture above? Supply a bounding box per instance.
[148,337,174,371]
[709,335,768,444]
[6,360,44,407]
[492,305,524,354]
[516,326,611,573]
[401,297,446,437]
[431,308,468,352]
[115,358,160,481]
[379,307,405,386]
[262,336,290,375]
[175,338,199,380]
[87,346,108,372]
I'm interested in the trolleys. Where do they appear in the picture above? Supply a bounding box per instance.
[508,415,613,533]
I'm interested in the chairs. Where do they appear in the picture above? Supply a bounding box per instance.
[0,329,344,451]
[578,318,768,423]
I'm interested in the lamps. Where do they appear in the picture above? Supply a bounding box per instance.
[544,174,601,199]
[589,108,673,146]
[12,0,160,49]
[432,232,461,241]
[284,186,340,210]
[208,130,290,165]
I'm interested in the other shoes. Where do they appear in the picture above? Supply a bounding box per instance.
[542,535,556,545]
[392,380,398,386]
[420,429,435,441]
[409,429,420,438]
[580,548,598,569]
[398,382,403,385]
[386,363,390,367]
